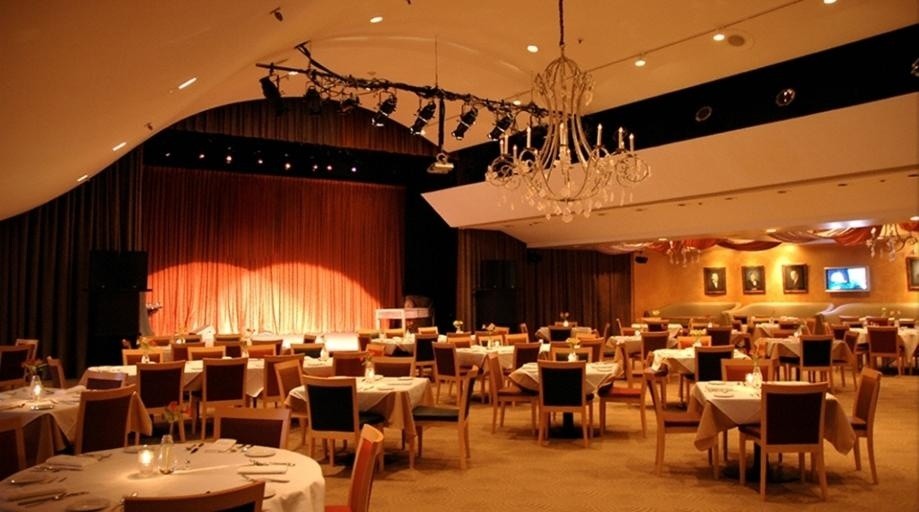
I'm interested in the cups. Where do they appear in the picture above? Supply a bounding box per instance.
[746,375,753,386]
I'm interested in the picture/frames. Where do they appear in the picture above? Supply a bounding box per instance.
[703,264,809,295]
[905,257,919,290]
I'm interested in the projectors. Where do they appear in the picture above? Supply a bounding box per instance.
[427,161,454,174]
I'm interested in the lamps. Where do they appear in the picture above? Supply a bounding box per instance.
[867,224,919,262]
[247,49,570,146]
[486,1,652,226]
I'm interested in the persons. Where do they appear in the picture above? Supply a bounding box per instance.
[785,270,805,291]
[829,271,865,290]
[743,270,762,291]
[708,271,721,290]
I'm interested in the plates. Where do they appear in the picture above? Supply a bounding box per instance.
[706,379,734,398]
[5,435,295,512]
[358,373,414,390]
[593,360,614,371]
[522,362,538,373]
[0,387,81,411]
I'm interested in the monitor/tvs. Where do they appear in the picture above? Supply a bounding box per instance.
[824,265,870,293]
